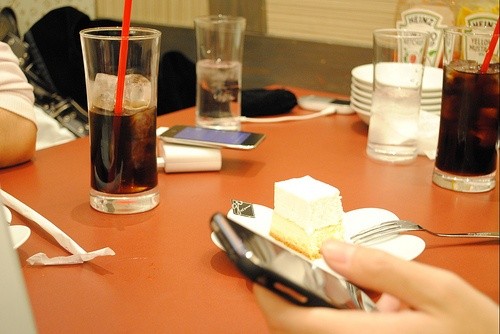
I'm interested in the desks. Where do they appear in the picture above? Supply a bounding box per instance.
[0,85,500,334]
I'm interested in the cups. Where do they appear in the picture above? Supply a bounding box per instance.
[366,28,430,164]
[79,26,162,215]
[431,25,500,194]
[193,14,246,132]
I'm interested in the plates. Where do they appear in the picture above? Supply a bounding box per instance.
[209,206,426,281]
[349,61,444,126]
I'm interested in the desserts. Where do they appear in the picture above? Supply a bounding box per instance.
[269,176,345,264]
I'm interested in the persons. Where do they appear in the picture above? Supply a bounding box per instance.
[0,39,40,169]
[252,244,500,333]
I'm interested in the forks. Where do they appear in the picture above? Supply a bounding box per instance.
[349,220,500,245]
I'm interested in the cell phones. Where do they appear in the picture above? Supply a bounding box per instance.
[209,212,376,312]
[297,94,355,114]
[159,125,265,149]
